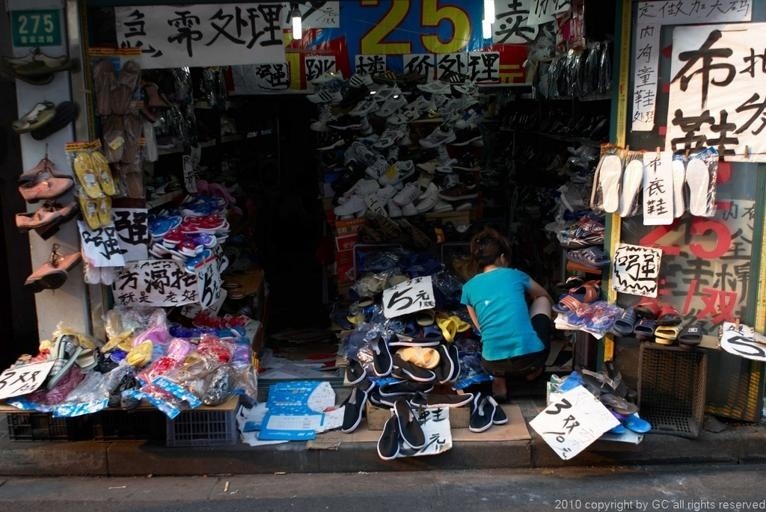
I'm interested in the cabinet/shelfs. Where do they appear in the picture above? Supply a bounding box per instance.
[409,91,611,285]
[219,260,269,354]
[141,99,272,210]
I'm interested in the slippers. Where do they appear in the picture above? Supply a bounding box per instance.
[10,309,258,411]
[550,369,652,434]
[73,60,145,230]
[551,216,611,312]
[334,217,471,337]
[563,301,705,349]
[148,180,243,275]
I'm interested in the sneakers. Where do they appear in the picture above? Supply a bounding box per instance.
[590,155,716,217]
[342,332,508,461]
[11,101,55,133]
[500,106,610,139]
[306,82,487,218]
[31,101,80,140]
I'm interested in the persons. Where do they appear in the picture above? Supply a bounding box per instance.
[459,227,555,403]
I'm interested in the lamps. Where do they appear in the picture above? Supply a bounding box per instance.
[284,0,307,41]
[482,17,492,40]
[484,0,495,24]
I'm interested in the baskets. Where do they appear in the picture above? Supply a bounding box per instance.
[706,347,761,422]
[638,343,707,438]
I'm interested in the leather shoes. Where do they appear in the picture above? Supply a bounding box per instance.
[16,202,78,236]
[35,205,80,241]
[18,178,74,204]
[24,263,68,293]
[18,159,73,185]
[54,252,82,272]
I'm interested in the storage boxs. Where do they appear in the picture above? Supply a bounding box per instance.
[320,180,366,299]
[366,390,471,431]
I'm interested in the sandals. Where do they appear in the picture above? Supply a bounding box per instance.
[2,48,82,85]
